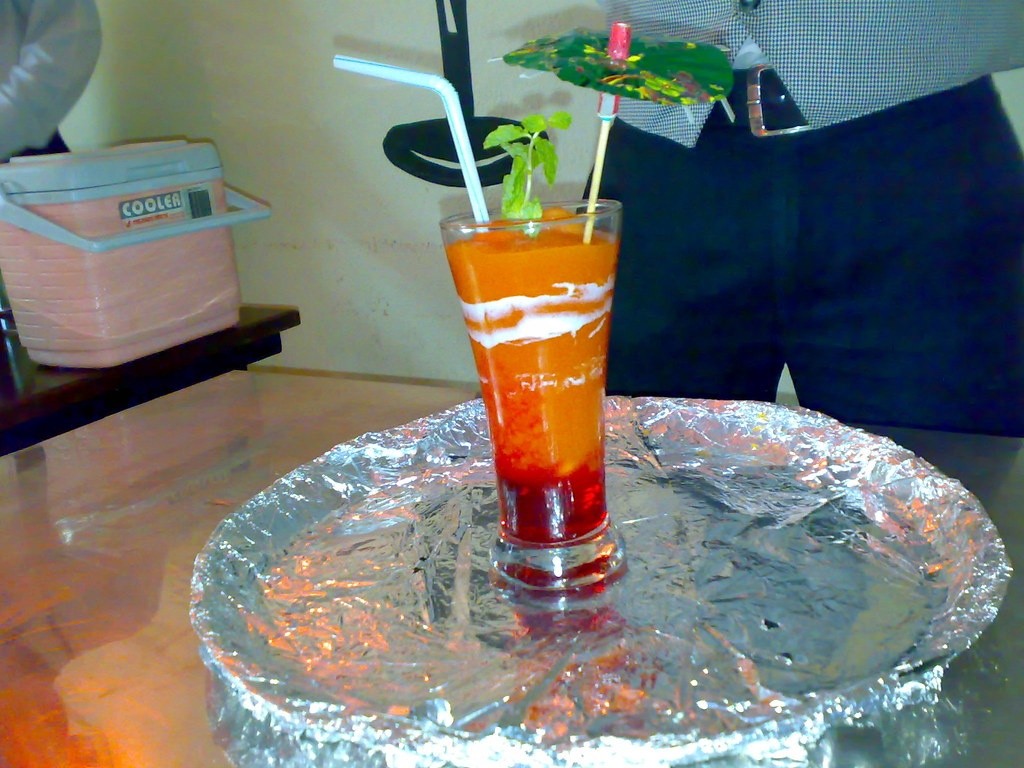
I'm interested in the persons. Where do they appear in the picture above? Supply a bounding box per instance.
[0,0,101,163]
[577,0,1023,437]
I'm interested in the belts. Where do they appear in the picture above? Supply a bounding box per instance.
[706,64,812,137]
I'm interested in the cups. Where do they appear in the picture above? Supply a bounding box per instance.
[439,200,628,591]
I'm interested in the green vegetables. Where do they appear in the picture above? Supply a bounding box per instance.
[481,109,572,234]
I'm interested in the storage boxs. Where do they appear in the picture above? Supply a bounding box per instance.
[0,139,272,369]
